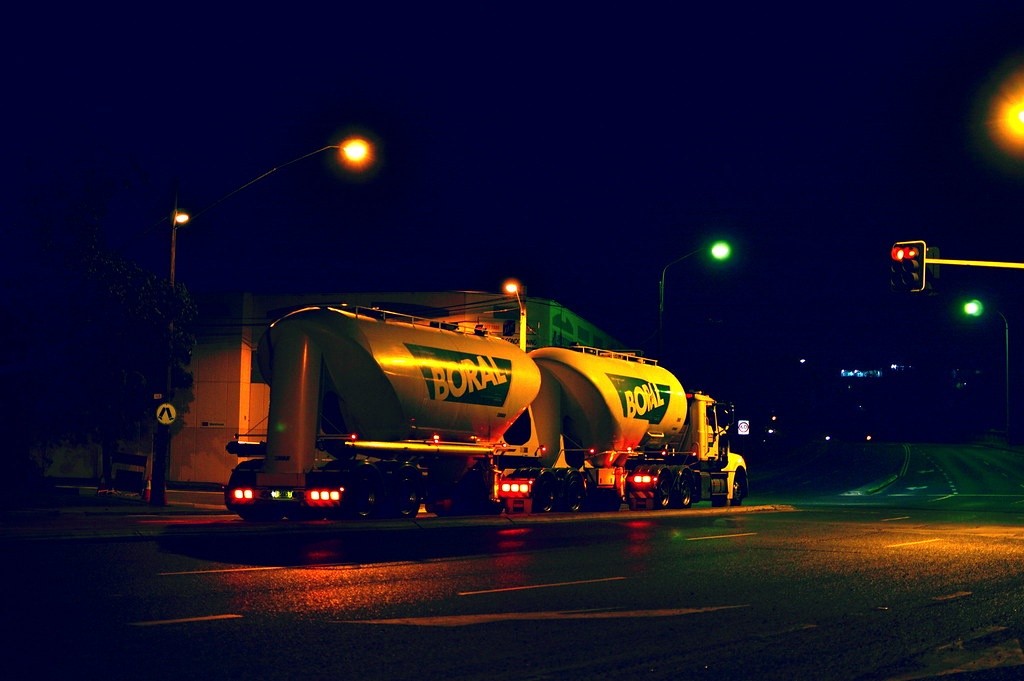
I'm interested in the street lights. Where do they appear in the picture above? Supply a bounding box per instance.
[166,139,369,403]
[964,300,1010,430]
[505,283,526,352]
[656,241,730,366]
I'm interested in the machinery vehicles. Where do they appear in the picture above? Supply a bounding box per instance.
[225,304,750,521]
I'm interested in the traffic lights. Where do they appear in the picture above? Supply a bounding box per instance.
[890,241,926,292]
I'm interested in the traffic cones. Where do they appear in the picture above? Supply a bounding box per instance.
[164,481,169,505]
[144,472,151,502]
